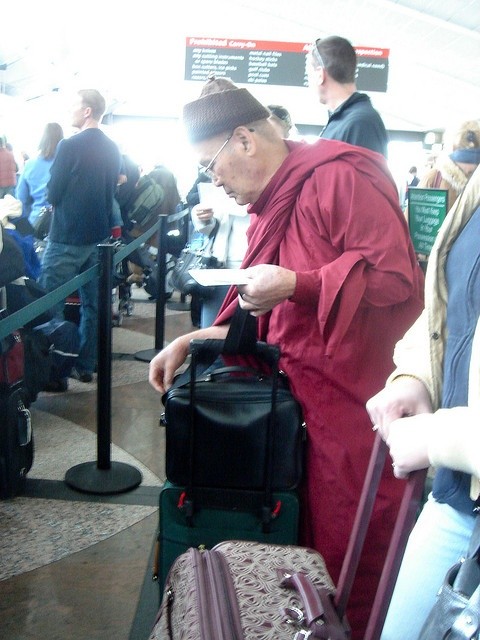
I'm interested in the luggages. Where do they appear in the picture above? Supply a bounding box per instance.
[159,340,299,608]
[147,417,426,640]
[2,388,33,501]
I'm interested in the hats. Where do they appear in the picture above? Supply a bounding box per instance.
[182,78,270,141]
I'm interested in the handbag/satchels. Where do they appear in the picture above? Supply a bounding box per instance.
[416,514,480,640]
[0,331,25,388]
[165,286,303,492]
[27,319,80,393]
[168,218,220,297]
[33,207,53,240]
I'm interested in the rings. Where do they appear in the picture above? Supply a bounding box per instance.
[370,423,381,432]
[391,462,395,468]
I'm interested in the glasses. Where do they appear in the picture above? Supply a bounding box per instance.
[198,126,253,176]
[309,38,324,66]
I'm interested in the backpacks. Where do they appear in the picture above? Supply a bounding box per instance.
[124,176,165,227]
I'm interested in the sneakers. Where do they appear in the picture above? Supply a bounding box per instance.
[68,367,93,382]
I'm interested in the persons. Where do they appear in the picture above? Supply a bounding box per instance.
[4,143,20,187]
[134,144,183,302]
[38,89,121,382]
[0,138,17,199]
[364,160,480,640]
[305,36,388,172]
[404,120,480,273]
[0,222,81,392]
[14,123,65,268]
[114,154,140,315]
[176,103,293,379]
[403,167,421,215]
[149,75,425,640]
